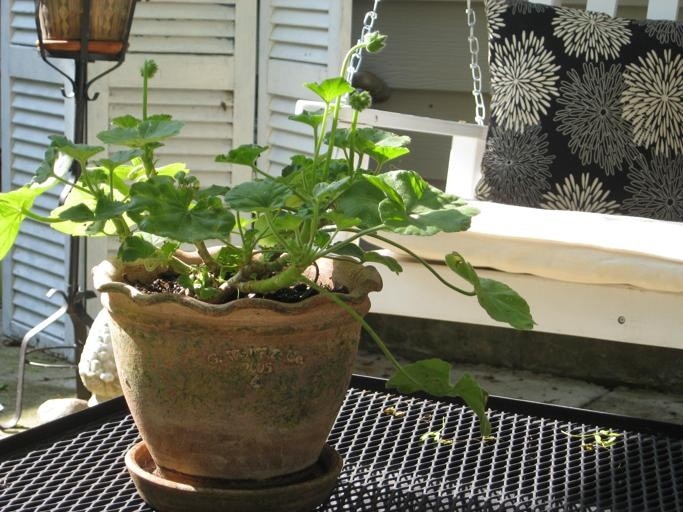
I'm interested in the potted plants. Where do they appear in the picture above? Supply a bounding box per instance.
[0,32,534,511]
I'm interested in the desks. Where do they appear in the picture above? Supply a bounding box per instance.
[0,353,683,511]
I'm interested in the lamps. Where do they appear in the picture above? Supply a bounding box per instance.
[13,1,141,426]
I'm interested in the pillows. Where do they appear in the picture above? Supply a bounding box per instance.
[470,0,680,224]
